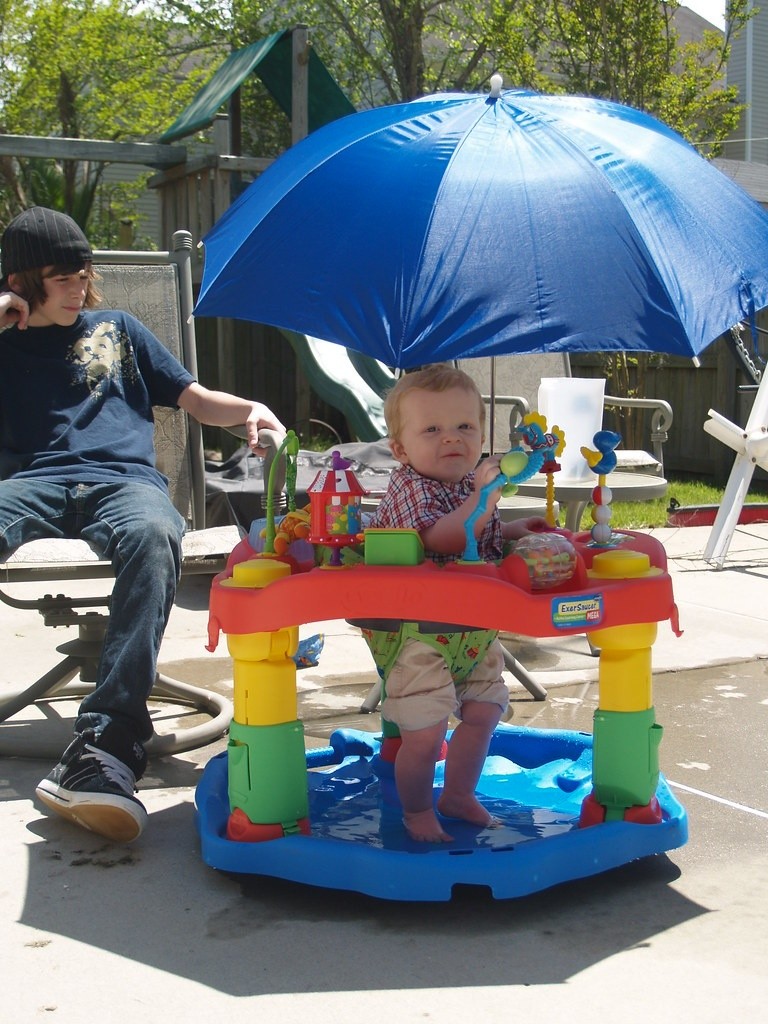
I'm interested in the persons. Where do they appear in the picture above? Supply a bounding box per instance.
[0,208,286,842]
[357,367,552,845]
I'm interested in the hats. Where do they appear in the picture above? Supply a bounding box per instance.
[0,206,94,285]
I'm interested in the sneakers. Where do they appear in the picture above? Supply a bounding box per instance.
[35,720,148,843]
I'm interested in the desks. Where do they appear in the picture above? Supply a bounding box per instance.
[357,490,559,715]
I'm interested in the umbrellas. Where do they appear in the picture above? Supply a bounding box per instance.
[187,75,768,455]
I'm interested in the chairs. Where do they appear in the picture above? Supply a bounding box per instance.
[0,231,287,757]
[702,363,768,571]
[450,352,671,659]
